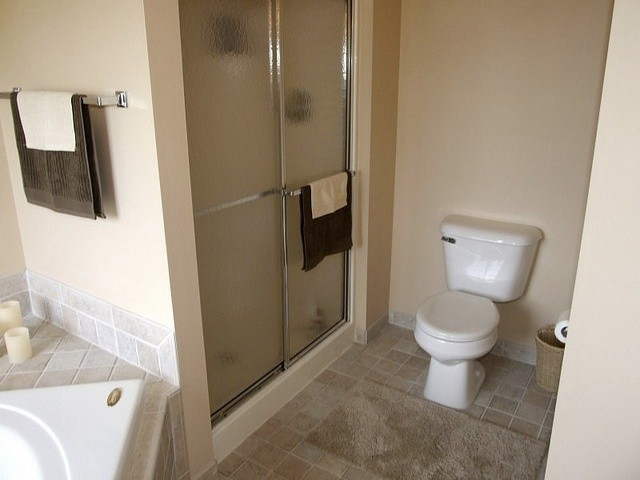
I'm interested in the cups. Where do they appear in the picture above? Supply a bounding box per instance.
[5,327,31,364]
[0,301,21,335]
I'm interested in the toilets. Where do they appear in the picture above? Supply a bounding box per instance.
[413,213,545,411]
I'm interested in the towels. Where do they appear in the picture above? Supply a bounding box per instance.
[307,172,348,220]
[10,93,106,220]
[16,91,76,152]
[299,171,352,272]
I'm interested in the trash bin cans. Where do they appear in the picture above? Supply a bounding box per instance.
[534,325,565,393]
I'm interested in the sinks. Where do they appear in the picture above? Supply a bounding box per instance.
[1,378,169,480]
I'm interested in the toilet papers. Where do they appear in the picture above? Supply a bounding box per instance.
[554,311,570,344]
